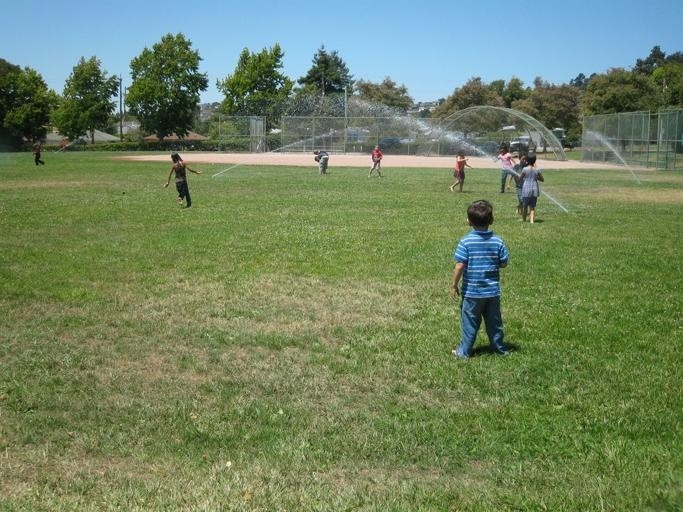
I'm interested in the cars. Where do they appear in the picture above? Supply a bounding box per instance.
[509,136,537,154]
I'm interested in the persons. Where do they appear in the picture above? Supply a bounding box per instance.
[368,145,383,178]
[507,154,527,216]
[33,143,45,166]
[164,152,201,209]
[450,199,514,359]
[494,142,515,193]
[450,153,474,193]
[314,149,329,175]
[516,154,544,224]
